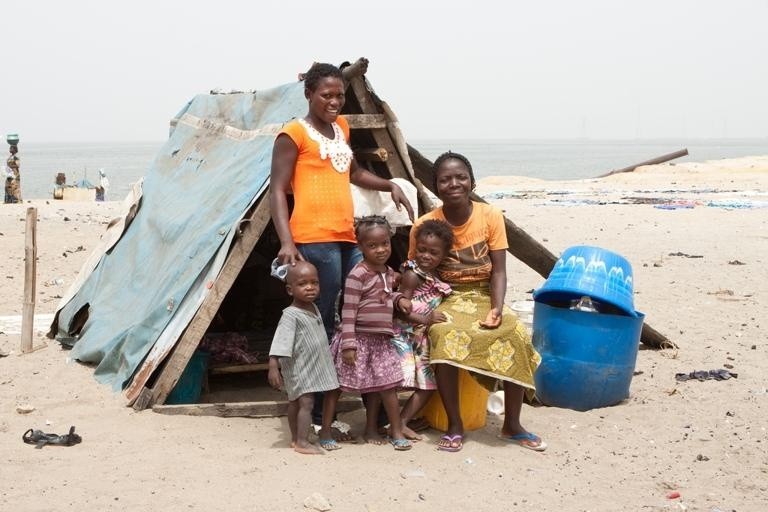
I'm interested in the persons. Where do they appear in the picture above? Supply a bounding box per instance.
[267,260,325,455]
[318,214,412,451]
[363,217,453,445]
[270,63,415,447]
[406,151,547,452]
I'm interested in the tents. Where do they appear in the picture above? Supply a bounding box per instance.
[45,55,435,419]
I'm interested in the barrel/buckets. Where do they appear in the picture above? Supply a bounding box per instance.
[156,346,211,405]
[421,365,491,432]
[531,299,646,412]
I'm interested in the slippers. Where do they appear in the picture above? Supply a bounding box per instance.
[310,421,357,443]
[406,417,430,431]
[438,432,462,451]
[319,439,342,450]
[389,437,412,450]
[497,429,547,450]
